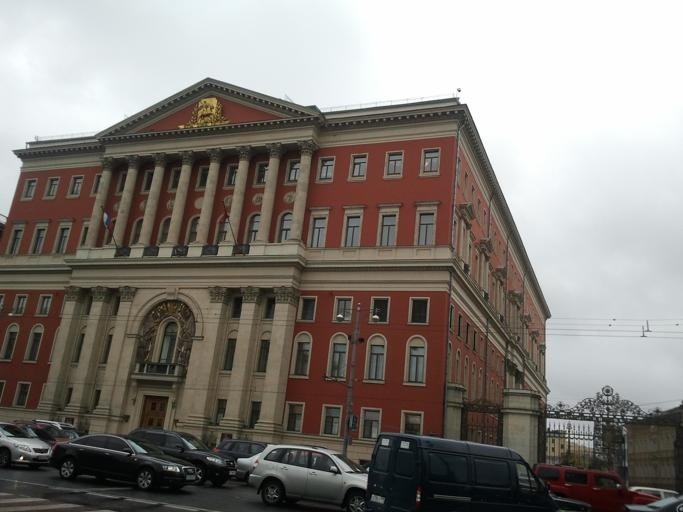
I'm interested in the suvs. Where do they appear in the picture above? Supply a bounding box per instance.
[213,439,269,463]
[125,427,235,489]
[247,443,367,512]
[7,417,80,446]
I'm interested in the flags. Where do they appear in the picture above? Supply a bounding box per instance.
[214,201,227,225]
[102,210,114,236]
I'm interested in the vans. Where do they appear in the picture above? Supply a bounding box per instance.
[364,430,592,512]
[532,461,683,511]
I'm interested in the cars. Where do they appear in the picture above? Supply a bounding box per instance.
[0,421,53,470]
[48,433,204,493]
[235,449,318,483]
